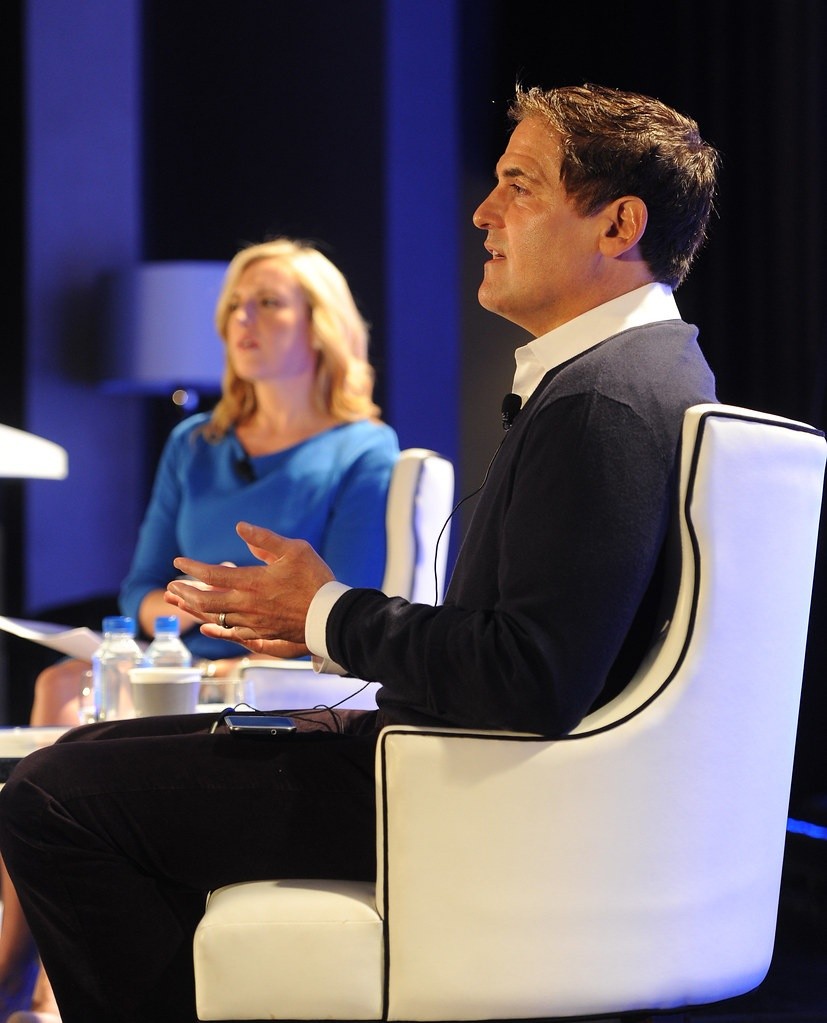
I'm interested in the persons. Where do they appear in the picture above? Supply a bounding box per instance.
[0,241,401,1023]
[0,88,718,1023]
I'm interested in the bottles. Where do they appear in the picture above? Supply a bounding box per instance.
[92,617,142,724]
[139,616,191,668]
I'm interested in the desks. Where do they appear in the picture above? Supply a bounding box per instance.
[0,725,79,784]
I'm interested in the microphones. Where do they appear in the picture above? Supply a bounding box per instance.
[500,393,522,425]
[235,461,254,483]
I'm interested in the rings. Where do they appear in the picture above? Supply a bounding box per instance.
[220,613,234,629]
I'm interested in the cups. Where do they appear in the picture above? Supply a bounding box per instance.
[77,668,97,728]
[124,667,201,718]
[197,678,255,713]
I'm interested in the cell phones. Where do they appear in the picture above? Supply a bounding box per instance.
[224,715,298,738]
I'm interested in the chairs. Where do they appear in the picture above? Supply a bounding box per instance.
[193,401,827,1023]
[238,447,454,709]
